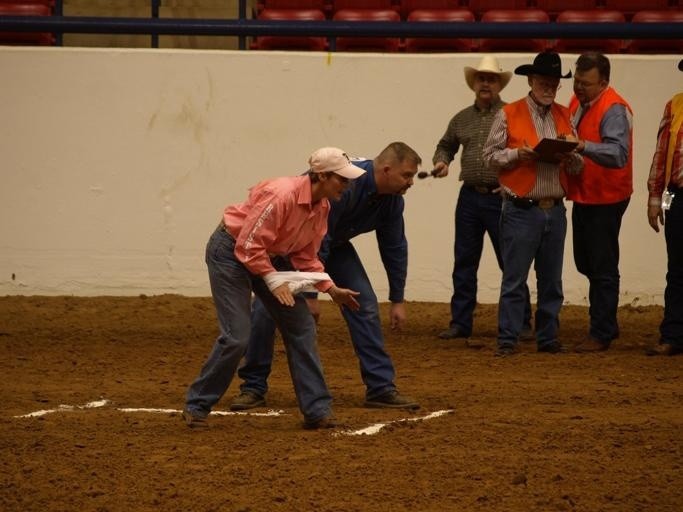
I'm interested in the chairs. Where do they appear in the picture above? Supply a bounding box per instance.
[0,1,683,55]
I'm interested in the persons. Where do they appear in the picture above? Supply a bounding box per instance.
[432,56,534,340]
[646,59,683,356]
[564,50,634,353]
[229,142,422,410]
[482,50,585,357]
[182,146,368,431]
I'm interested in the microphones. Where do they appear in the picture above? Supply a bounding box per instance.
[418,169,441,178]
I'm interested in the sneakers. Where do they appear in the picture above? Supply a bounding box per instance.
[436,321,473,340]
[177,407,212,434]
[494,338,522,358]
[518,322,535,343]
[227,389,269,411]
[302,412,344,430]
[361,387,422,410]
[645,337,683,355]
[574,333,620,353]
[536,333,570,354]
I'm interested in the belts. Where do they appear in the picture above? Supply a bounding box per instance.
[463,181,500,196]
[504,193,563,209]
[219,219,236,245]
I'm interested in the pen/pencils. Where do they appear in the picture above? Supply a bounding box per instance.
[522,139,530,149]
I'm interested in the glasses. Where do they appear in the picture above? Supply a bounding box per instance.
[418,169,444,179]
[532,76,564,92]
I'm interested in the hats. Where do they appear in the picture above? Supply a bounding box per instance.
[462,55,513,93]
[306,147,368,181]
[513,51,574,80]
